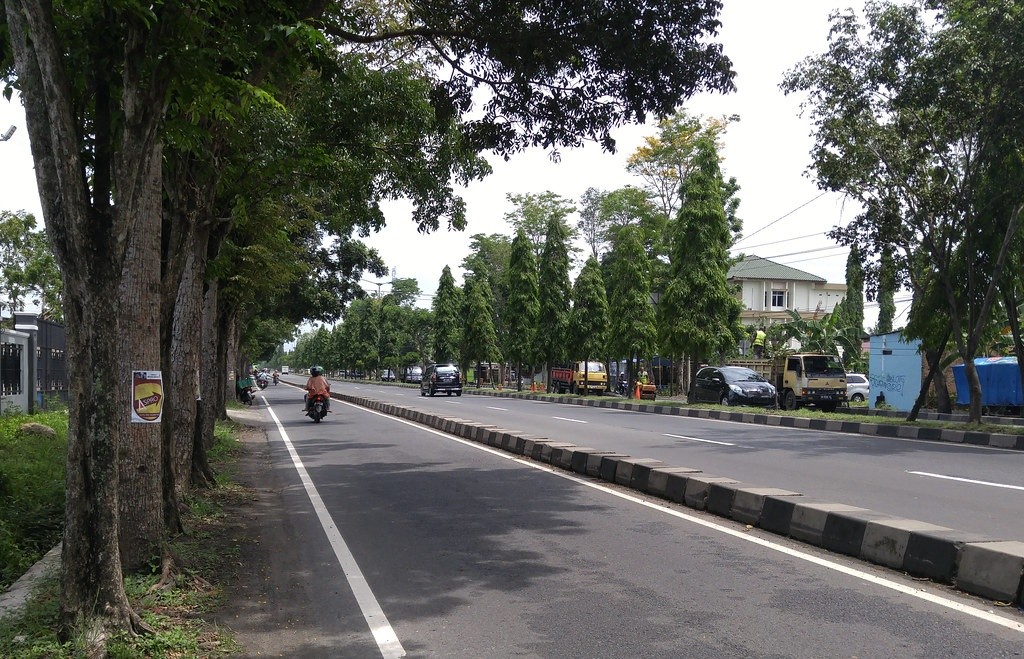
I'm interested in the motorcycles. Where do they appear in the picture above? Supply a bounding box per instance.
[304,383,332,422]
[240,383,256,406]
[252,373,280,391]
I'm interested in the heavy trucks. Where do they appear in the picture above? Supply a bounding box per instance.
[551,360,608,396]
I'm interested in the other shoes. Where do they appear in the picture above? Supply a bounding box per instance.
[327,410,331,412]
[302,408,307,411]
[305,413,309,416]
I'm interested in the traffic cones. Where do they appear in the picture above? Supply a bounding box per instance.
[635,385,641,401]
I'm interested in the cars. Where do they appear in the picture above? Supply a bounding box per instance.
[686,365,779,408]
[380,370,396,382]
[338,369,365,378]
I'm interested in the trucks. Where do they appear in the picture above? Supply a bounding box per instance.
[400,366,423,384]
[727,351,848,414]
[281,366,289,375]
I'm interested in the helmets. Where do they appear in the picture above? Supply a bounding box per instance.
[310,367,319,377]
[316,366,323,375]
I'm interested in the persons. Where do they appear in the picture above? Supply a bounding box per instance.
[272,370,279,379]
[253,369,258,374]
[236,369,239,399]
[257,370,267,379]
[750,328,766,358]
[638,367,648,384]
[618,371,624,390]
[875,391,885,408]
[302,366,331,412]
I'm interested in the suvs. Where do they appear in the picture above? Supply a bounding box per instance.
[420,363,463,396]
[820,373,870,403]
[474,363,517,384]
